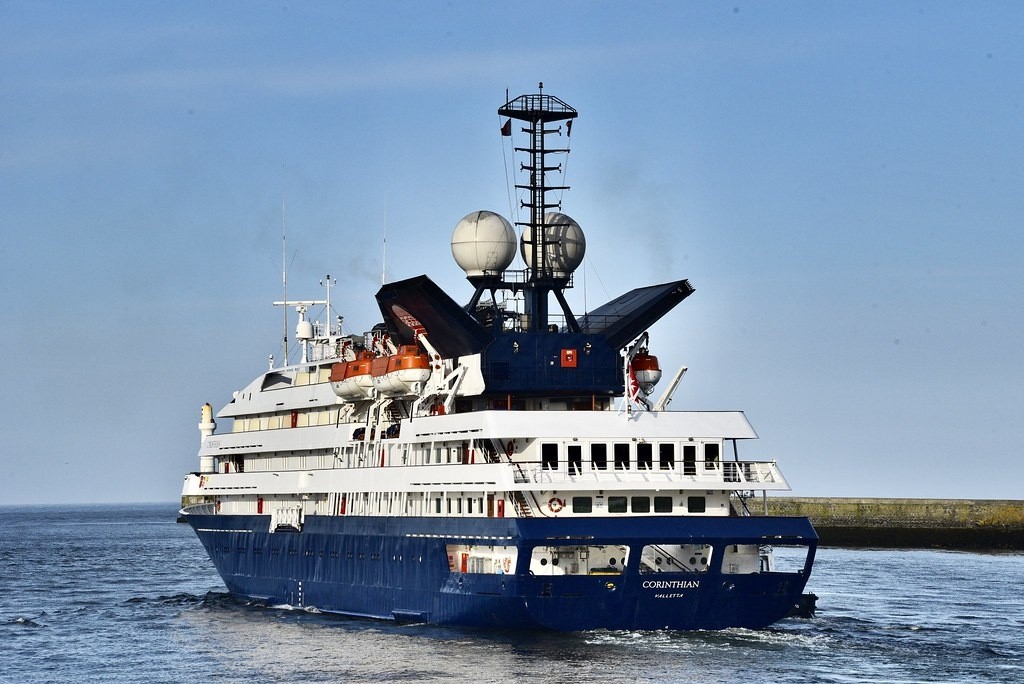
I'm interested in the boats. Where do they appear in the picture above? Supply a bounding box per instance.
[178,82,818,633]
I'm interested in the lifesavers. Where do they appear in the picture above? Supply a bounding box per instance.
[507,441,514,456]
[548,497,563,512]
